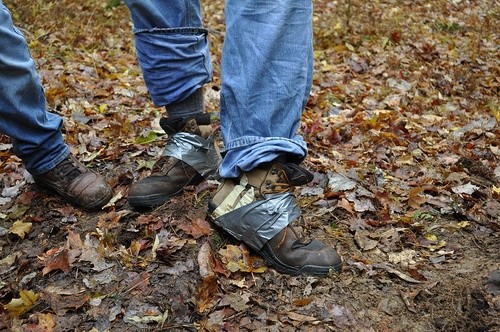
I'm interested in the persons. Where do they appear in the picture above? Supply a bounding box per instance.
[128,0,343,274]
[0,0,113,209]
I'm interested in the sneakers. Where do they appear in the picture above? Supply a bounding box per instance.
[32,153,111,211]
[205,157,342,278]
[128,114,222,207]
